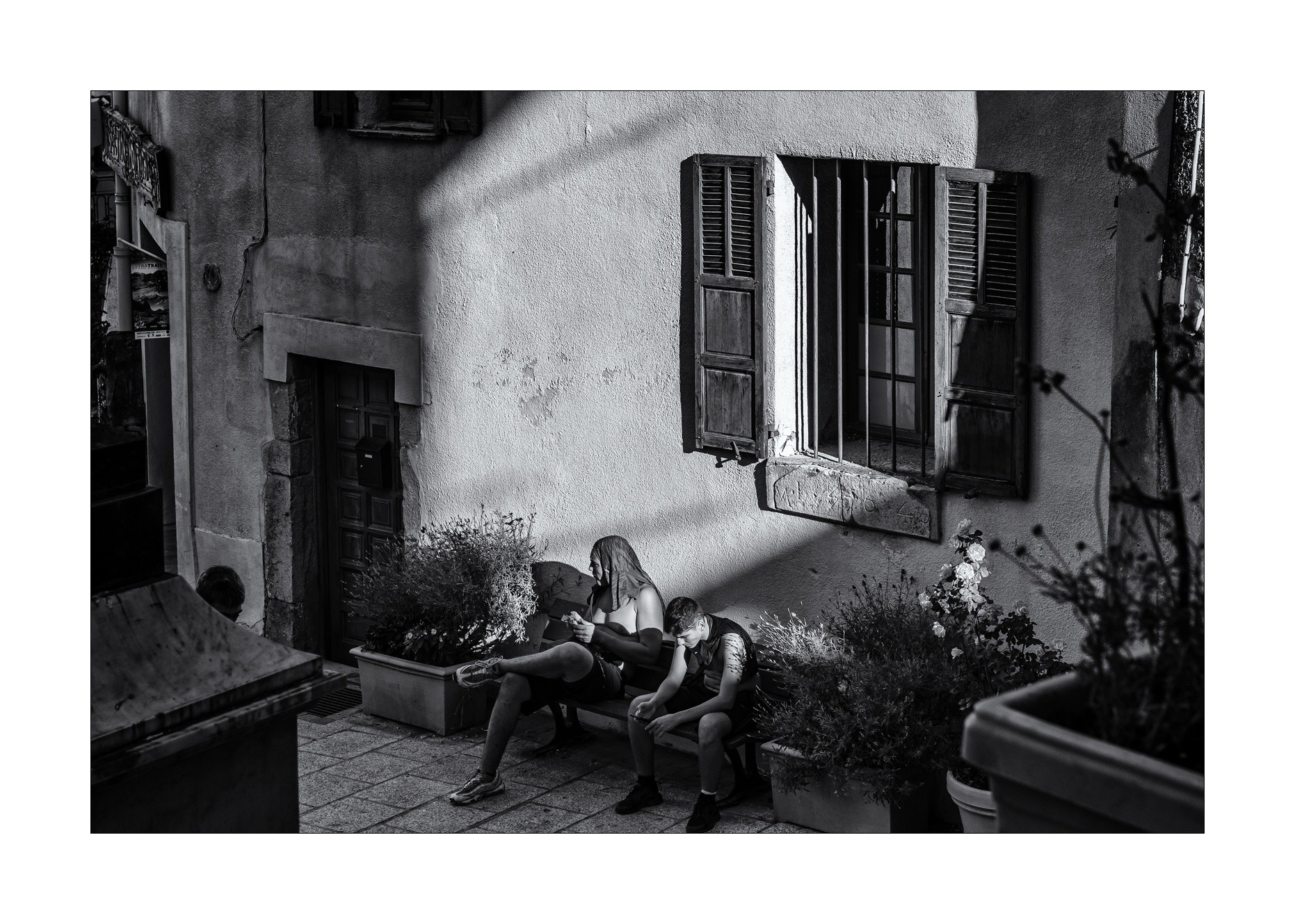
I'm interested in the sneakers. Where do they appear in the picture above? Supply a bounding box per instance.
[616,775,664,814]
[454,657,503,689]
[684,793,721,832]
[450,767,506,806]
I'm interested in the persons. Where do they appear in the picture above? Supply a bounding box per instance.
[193,565,246,621]
[616,596,762,833]
[450,536,666,805]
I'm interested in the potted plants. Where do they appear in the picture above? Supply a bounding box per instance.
[960,139,1205,834]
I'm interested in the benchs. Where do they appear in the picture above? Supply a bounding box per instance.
[491,597,801,811]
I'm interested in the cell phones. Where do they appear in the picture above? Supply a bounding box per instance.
[629,713,652,723]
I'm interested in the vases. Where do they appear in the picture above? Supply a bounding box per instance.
[944,769,996,833]
[348,644,487,736]
[760,734,901,833]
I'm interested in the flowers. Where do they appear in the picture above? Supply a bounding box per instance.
[338,505,584,669]
[748,519,1073,790]
